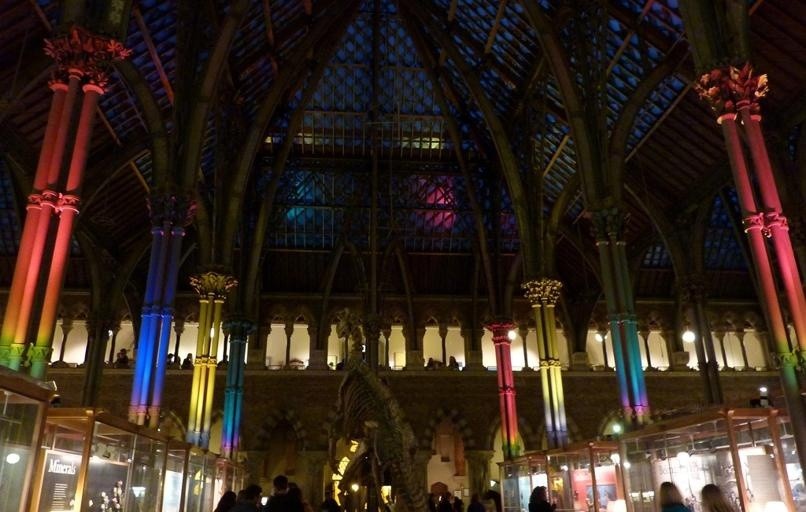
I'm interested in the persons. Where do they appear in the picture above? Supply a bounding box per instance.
[527,483,556,511]
[213,476,312,511]
[658,480,694,512]
[317,488,342,511]
[428,489,488,511]
[703,482,734,511]
[112,346,195,370]
